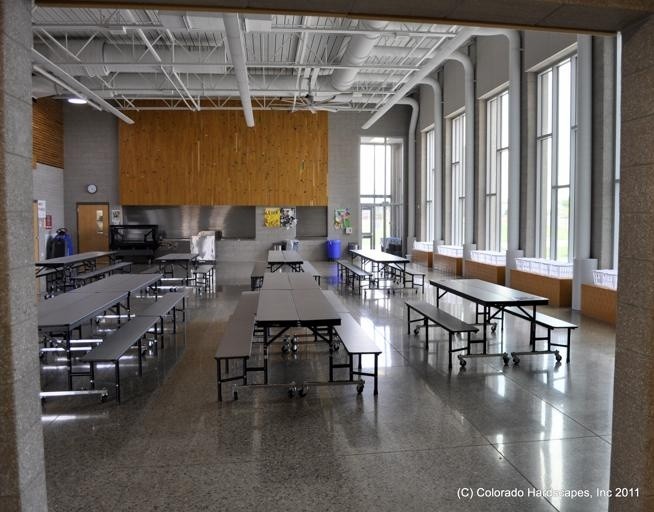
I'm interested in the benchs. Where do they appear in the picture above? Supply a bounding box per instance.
[35,249,579,401]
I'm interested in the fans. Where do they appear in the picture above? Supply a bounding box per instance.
[287,80,337,114]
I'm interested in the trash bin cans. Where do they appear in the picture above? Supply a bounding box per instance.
[327,240,342,261]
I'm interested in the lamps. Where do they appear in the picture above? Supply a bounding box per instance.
[68,97,87,105]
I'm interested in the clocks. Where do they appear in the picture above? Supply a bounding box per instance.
[87,184,97,194]
